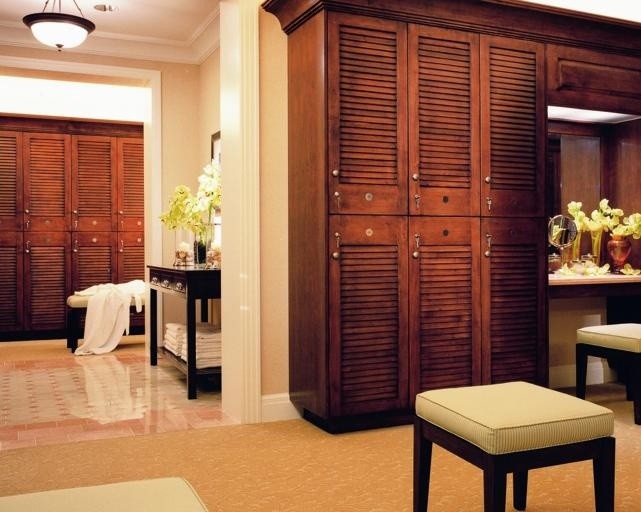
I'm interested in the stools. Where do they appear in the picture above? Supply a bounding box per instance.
[411,378,617,512]
[572,319,641,424]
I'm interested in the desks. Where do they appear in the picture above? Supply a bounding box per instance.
[547,271,641,299]
[145,263,222,402]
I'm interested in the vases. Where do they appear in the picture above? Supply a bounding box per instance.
[607,236,633,274]
[192,240,207,266]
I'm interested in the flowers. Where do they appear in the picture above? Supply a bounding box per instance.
[586,198,625,233]
[612,210,641,241]
[162,158,221,248]
[567,199,590,234]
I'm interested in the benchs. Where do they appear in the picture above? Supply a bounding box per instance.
[65,292,145,354]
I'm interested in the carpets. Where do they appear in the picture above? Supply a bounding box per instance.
[0,476,211,512]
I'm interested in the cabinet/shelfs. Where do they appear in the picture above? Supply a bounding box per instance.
[262,1,641,436]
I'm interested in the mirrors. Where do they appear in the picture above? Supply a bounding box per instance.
[548,123,641,273]
[547,213,580,275]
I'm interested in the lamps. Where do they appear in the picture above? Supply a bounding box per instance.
[21,0,98,54]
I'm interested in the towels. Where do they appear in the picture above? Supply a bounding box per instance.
[74,279,146,353]
[161,321,222,371]
